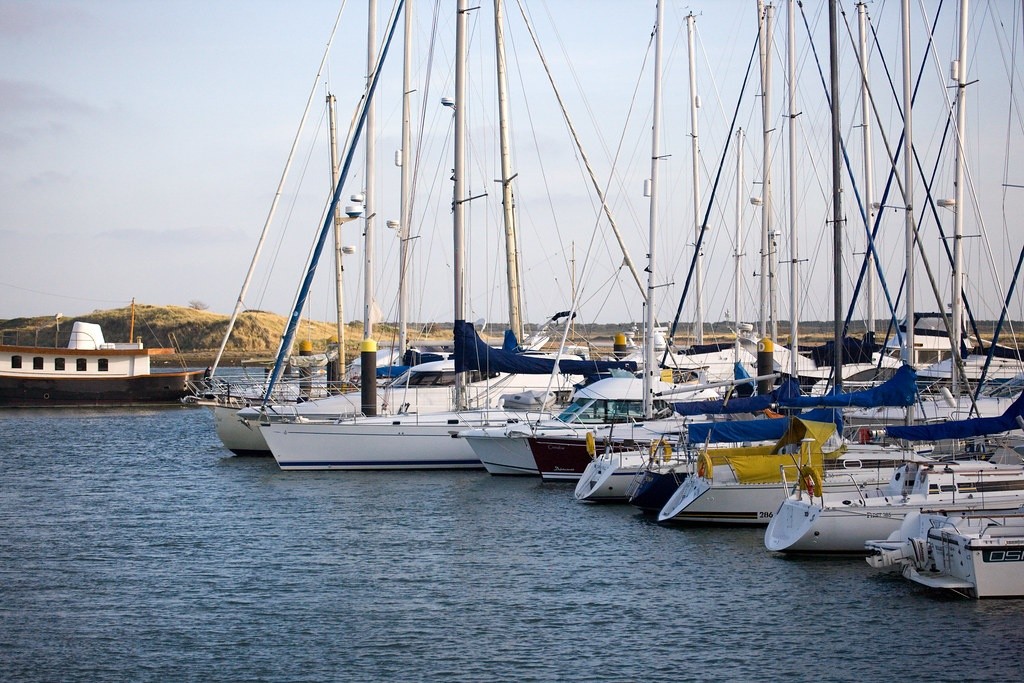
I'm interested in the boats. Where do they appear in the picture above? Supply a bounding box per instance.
[203,1,1023,599]
[0,282,206,401]
